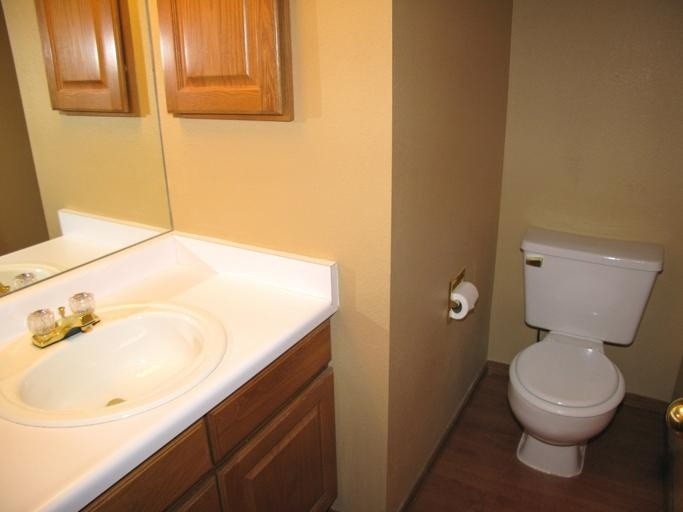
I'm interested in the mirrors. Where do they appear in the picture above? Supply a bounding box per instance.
[0,0,173,305]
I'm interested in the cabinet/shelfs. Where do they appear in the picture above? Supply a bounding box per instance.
[75,316,337,511]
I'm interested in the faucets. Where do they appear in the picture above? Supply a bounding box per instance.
[0,272,37,296]
[26,291,101,349]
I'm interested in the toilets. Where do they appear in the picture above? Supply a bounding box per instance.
[506,226,665,479]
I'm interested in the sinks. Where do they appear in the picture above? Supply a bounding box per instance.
[0,263,61,297]
[0,299,228,428]
